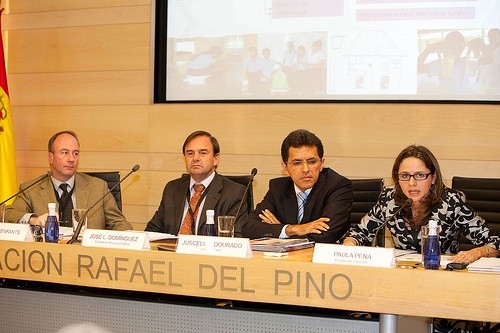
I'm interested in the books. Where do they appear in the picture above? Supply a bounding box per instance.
[467,257,500,273]
[249,237,314,252]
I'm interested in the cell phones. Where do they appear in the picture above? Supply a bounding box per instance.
[447,262,469,269]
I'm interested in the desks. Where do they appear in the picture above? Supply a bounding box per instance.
[0,222,500,333]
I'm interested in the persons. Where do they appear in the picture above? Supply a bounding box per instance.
[240,129,351,244]
[247,38,327,99]
[342,145,500,263]
[0,131,133,231]
[144,131,248,237]
[417,29,500,100]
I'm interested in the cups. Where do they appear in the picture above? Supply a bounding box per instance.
[72,209,88,240]
[421,225,440,265]
[218,216,235,238]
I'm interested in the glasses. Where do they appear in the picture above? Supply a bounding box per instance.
[397,172,431,181]
[286,159,322,166]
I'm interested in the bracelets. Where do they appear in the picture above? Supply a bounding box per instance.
[484,247,491,258]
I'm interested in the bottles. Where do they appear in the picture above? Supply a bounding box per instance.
[44,202,59,243]
[203,210,217,236]
[423,219,441,270]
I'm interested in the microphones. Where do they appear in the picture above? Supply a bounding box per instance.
[0,171,52,206]
[66,164,140,244]
[227,167,258,237]
[372,198,413,248]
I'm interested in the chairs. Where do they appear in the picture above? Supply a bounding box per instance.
[182,173,254,215]
[350,177,386,247]
[452,176,500,238]
[83,171,122,213]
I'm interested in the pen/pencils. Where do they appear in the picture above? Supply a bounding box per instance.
[57,220,69,223]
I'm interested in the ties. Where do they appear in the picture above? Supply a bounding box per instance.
[297,192,309,225]
[60,184,74,227]
[178,184,205,235]
